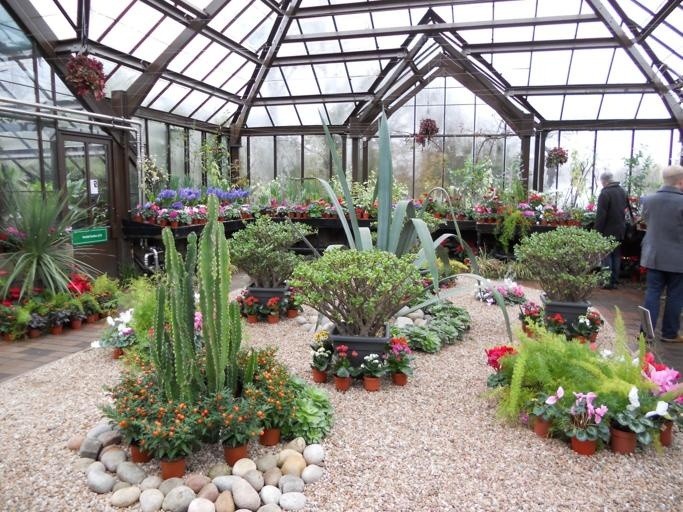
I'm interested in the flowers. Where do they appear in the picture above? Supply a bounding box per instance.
[483,340,683,445]
[0,223,125,340]
[518,301,605,344]
[308,323,419,378]
[64,54,107,101]
[95,343,300,460]
[236,290,303,315]
[416,118,439,143]
[545,146,569,168]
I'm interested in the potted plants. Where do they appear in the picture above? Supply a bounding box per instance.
[287,248,429,377]
[512,224,622,340]
[227,210,318,309]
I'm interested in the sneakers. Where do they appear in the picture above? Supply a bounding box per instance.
[659,333,683,343]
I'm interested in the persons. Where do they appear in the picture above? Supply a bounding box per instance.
[595,170,631,292]
[638,162,682,343]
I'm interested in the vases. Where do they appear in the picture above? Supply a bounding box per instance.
[364,375,380,392]
[610,429,636,454]
[333,375,351,392]
[571,437,598,456]
[247,313,257,324]
[313,369,326,383]
[269,315,279,324]
[160,458,186,480]
[395,374,407,386]
